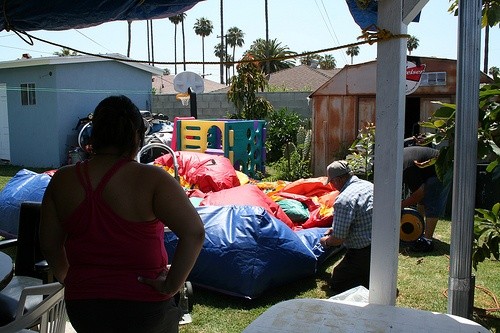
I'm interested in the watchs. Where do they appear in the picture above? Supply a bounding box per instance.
[323,238,331,249]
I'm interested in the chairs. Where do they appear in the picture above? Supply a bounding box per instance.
[1,201,61,333]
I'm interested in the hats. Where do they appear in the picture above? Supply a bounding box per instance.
[323,160,351,186]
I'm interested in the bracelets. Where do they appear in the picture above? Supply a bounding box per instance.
[163,279,178,297]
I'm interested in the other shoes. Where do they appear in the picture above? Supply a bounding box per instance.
[408,237,434,254]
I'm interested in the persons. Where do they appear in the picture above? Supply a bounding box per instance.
[37,93,205,333]
[319,159,400,298]
[369,145,454,254]
